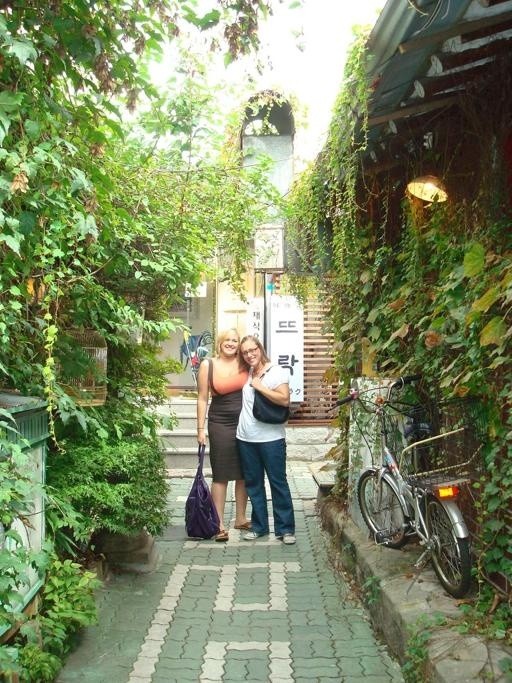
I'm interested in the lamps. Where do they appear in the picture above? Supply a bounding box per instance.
[406,169,448,204]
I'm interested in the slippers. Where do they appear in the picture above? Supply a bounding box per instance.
[234,521,252,529]
[216,530,228,541]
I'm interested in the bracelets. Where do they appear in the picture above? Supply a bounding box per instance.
[197,428,204,429]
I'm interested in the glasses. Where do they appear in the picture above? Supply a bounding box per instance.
[241,347,259,355]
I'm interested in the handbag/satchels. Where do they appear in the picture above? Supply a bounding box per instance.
[185,473,220,539]
[253,388,289,424]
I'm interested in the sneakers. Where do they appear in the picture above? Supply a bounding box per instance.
[282,532,296,544]
[243,531,260,540]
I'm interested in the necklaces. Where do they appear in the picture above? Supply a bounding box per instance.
[253,364,264,375]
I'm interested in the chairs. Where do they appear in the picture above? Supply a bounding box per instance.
[178,335,209,390]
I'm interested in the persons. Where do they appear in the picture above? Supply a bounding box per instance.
[195,324,259,543]
[232,335,302,546]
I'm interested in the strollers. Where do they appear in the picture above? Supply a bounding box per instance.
[180,331,213,387]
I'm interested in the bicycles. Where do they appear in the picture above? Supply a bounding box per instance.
[325,375,483,598]
